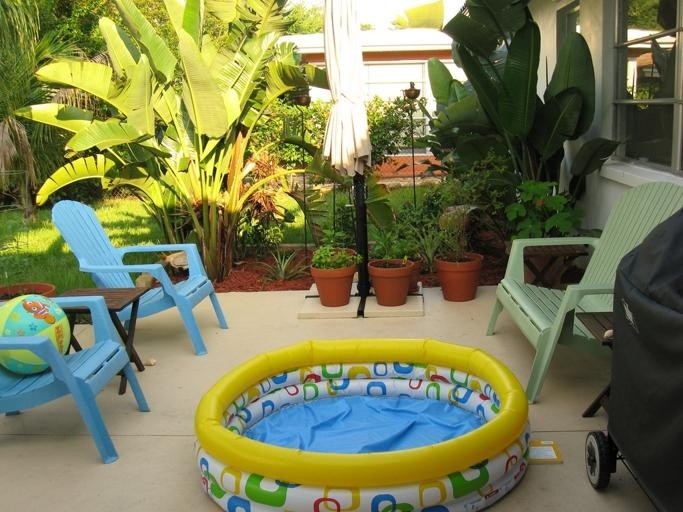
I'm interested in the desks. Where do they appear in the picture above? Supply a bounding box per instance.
[54,287,146,394]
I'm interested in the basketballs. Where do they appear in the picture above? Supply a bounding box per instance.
[0,293,70,374]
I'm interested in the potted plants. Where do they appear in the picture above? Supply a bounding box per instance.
[1,244,53,301]
[303,144,513,306]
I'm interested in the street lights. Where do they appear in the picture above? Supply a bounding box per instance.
[291,95,311,279]
[401,82,425,211]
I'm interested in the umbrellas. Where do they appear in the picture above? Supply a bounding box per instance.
[321,0,375,294]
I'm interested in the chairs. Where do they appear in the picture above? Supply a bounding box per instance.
[51,200,228,357]
[2,296,152,464]
[485,180,683,405]
